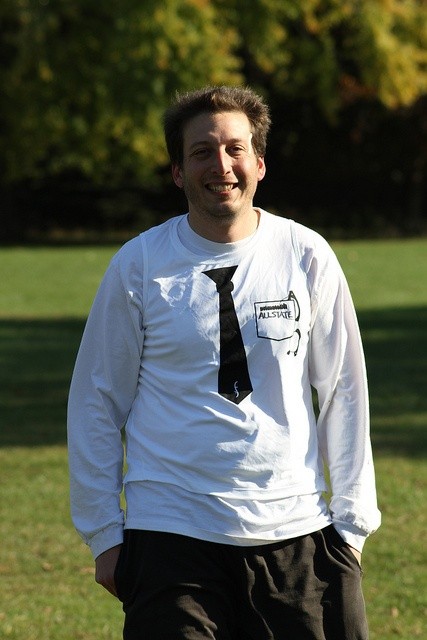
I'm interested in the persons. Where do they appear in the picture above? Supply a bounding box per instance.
[65,87,384,640]
